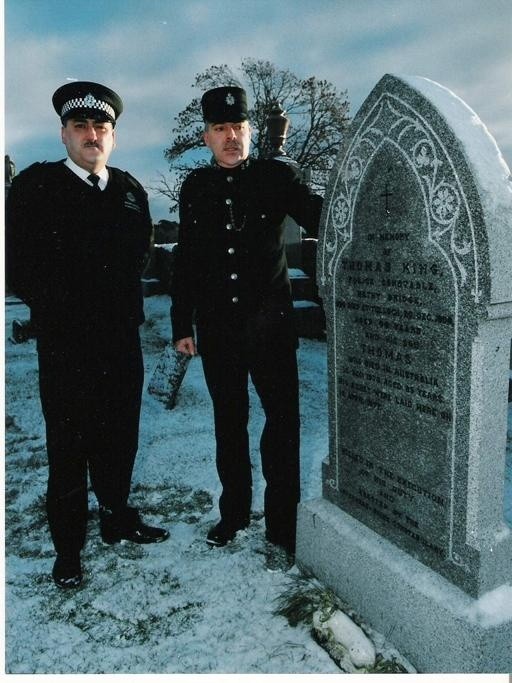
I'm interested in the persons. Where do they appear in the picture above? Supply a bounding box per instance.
[166,86,327,558]
[4,79,168,591]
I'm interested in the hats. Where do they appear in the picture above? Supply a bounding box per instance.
[52,82,122,127]
[201,87,249,123]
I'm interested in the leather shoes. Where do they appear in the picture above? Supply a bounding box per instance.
[206,515,249,546]
[53,552,81,588]
[104,521,170,543]
